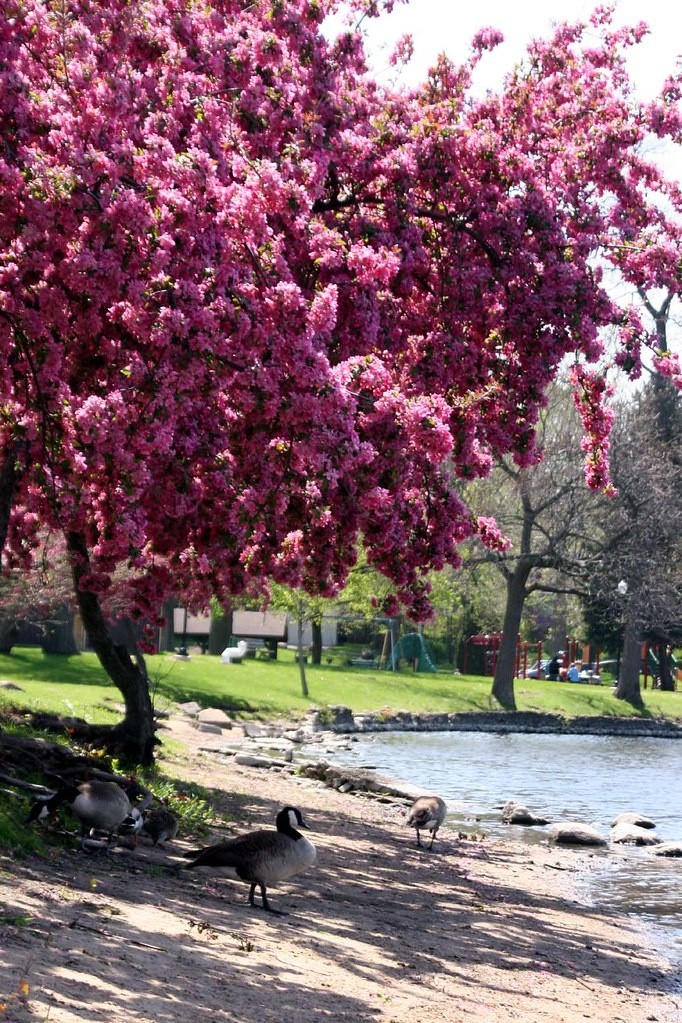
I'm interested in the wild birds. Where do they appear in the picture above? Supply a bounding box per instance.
[182,806,318,916]
[406,795,446,852]
[24,776,180,850]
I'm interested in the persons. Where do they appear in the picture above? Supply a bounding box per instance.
[566,662,581,683]
[550,655,559,680]
